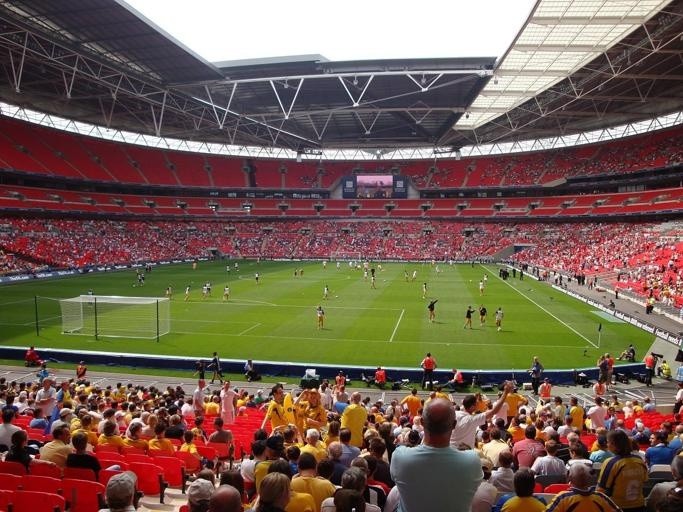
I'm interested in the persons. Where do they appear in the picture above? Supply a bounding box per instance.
[389,338,682,511]
[1,214,208,289]
[413,150,549,186]
[0,339,409,511]
[495,307,503,330]
[548,138,682,174]
[165,281,232,302]
[208,218,426,291]
[317,307,326,330]
[321,285,328,300]
[479,279,484,296]
[582,223,683,317]
[428,298,438,323]
[418,221,621,291]
[463,305,476,329]
[479,303,488,327]
[422,283,427,299]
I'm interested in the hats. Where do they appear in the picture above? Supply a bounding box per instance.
[304,388,321,400]
[269,383,283,396]
[106,469,137,500]
[186,478,215,506]
